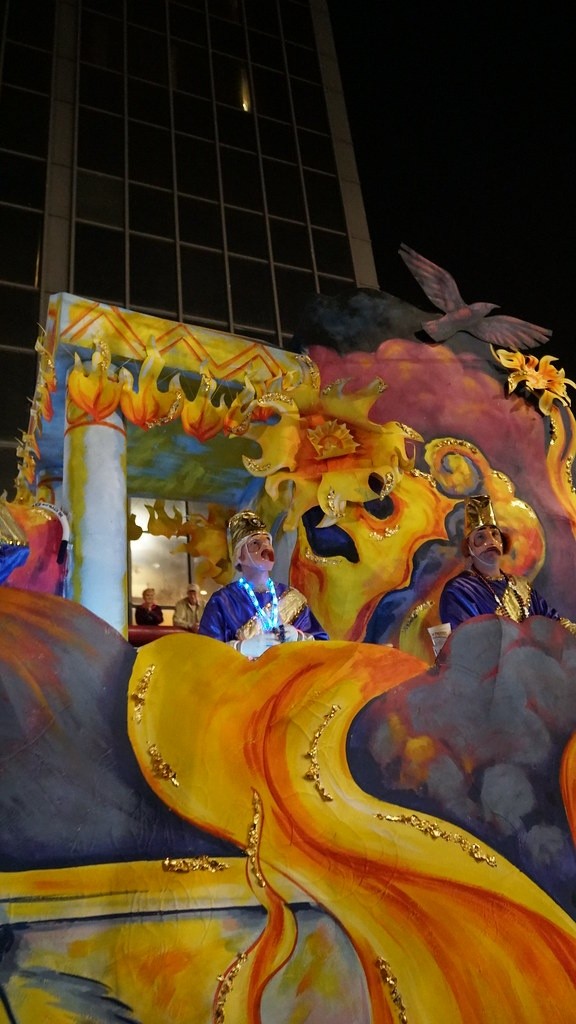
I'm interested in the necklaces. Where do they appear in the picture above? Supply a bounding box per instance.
[472,568,526,622]
[238,577,278,630]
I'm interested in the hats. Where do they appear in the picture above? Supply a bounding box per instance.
[187,584,199,591]
[464,496,497,539]
[227,511,272,566]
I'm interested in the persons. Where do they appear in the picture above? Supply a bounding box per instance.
[194,510,329,659]
[172,584,207,632]
[439,496,576,635]
[135,588,163,626]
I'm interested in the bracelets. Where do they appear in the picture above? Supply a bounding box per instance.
[279,625,285,642]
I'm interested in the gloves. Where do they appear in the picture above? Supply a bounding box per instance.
[272,624,298,643]
[241,633,281,658]
